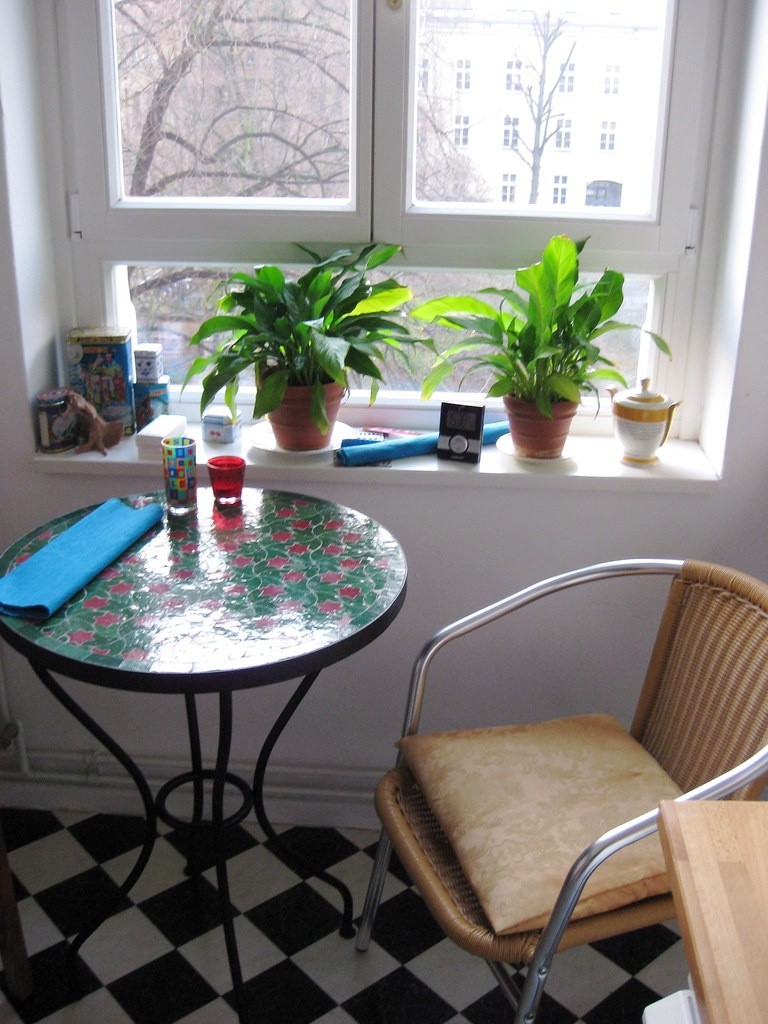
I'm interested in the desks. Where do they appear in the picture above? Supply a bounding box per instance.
[1,485,408,1024]
[656,798,766,1024]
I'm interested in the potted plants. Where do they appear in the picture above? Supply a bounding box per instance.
[180,240,440,451]
[411,230,674,459]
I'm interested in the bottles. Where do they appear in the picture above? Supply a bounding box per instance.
[36,387,81,453]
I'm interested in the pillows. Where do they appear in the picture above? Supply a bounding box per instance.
[399,712,684,935]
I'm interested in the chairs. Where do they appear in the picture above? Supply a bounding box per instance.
[355,559,768,1024]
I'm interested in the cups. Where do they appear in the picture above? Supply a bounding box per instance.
[206,455,246,508]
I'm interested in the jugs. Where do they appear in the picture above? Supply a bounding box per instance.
[605,377,681,467]
